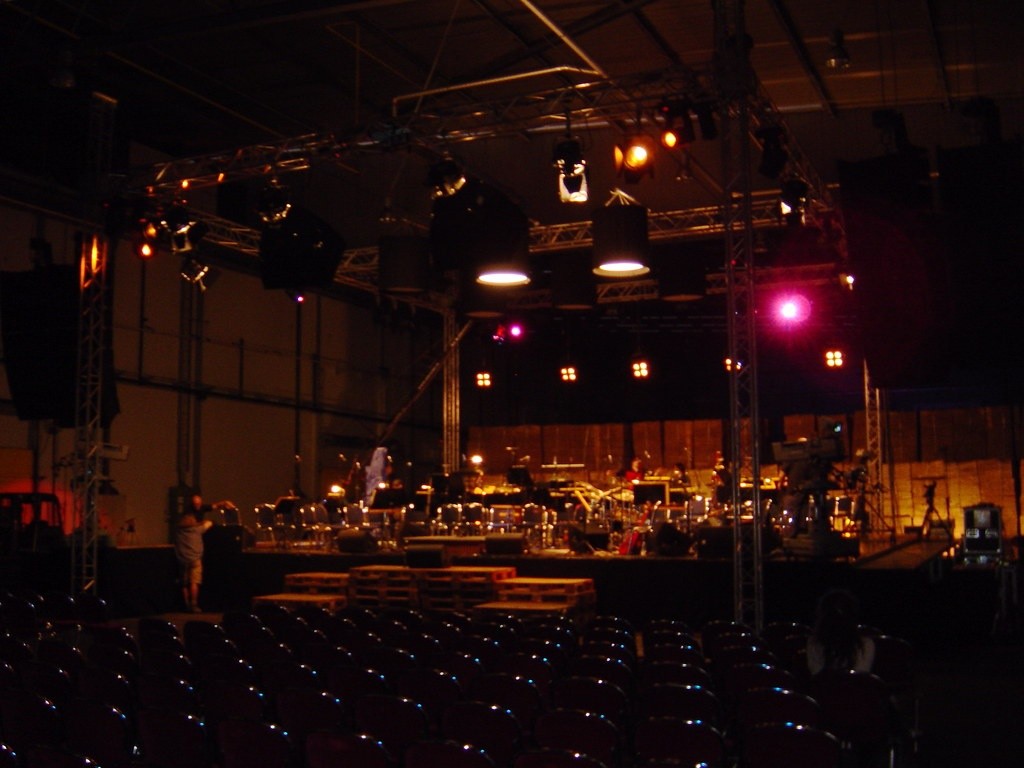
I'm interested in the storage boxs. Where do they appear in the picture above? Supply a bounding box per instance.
[963,508,1002,555]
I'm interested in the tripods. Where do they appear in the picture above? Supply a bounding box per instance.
[917,500,953,540]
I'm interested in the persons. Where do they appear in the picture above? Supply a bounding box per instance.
[175,415,879,671]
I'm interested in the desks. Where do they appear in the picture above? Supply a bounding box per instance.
[252,592,348,612]
[472,598,573,617]
[286,572,349,594]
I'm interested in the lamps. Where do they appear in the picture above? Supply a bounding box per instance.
[552,136,594,194]
[474,204,533,289]
[590,194,652,279]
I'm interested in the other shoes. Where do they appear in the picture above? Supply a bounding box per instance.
[188,605,202,613]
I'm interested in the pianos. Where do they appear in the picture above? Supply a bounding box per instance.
[540,463,586,469]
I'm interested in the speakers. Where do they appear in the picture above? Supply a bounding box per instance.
[484,532,531,555]
[694,526,735,558]
[406,545,452,569]
[43,263,120,429]
[203,524,254,549]
[569,526,609,552]
[741,522,780,554]
[336,529,378,553]
[646,522,693,556]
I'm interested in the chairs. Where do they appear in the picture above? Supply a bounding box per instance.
[217,494,856,556]
[0,604,927,768]
